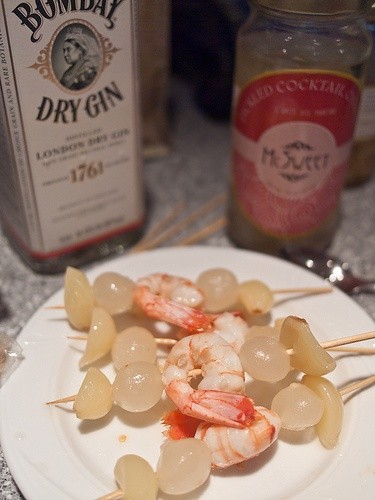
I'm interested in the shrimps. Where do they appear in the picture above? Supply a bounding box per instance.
[189,312,249,355]
[163,333,256,429]
[160,406,281,471]
[133,274,210,330]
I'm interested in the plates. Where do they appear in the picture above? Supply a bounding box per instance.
[0,243,375,499]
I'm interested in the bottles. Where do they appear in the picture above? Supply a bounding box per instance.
[1,0,149,275]
[339,15,375,187]
[226,0,369,261]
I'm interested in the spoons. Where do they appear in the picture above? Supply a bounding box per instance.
[290,258,374,295]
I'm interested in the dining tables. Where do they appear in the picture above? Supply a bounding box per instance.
[0,105,374,499]
